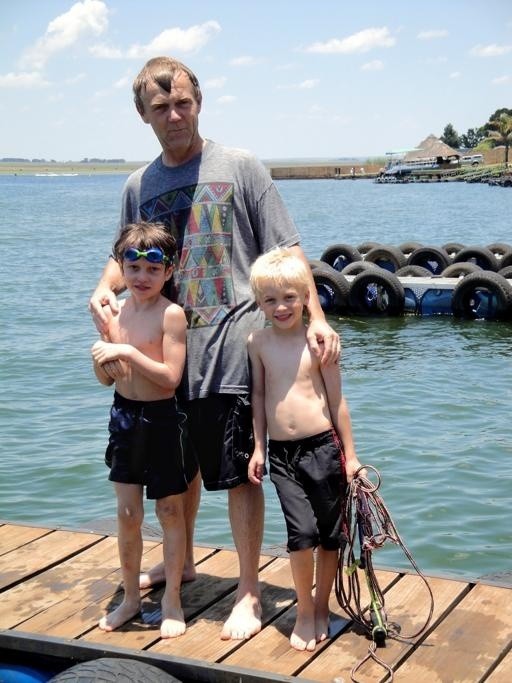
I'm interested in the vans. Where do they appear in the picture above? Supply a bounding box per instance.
[459,156,483,167]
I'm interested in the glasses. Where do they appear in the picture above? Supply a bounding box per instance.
[122,247,173,263]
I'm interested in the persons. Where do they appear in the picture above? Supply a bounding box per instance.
[90,221,190,640]
[246,247,368,652]
[88,57,341,641]
